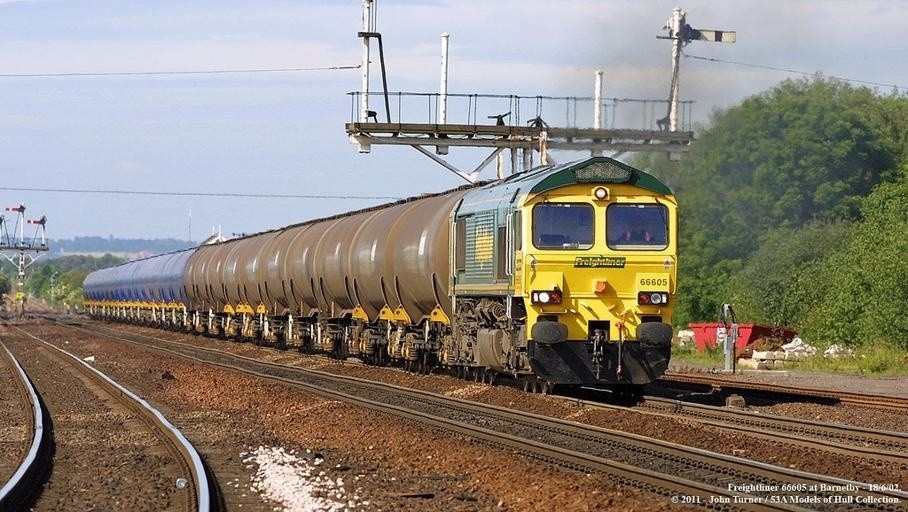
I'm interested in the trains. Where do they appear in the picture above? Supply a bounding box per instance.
[81,156,682,398]
